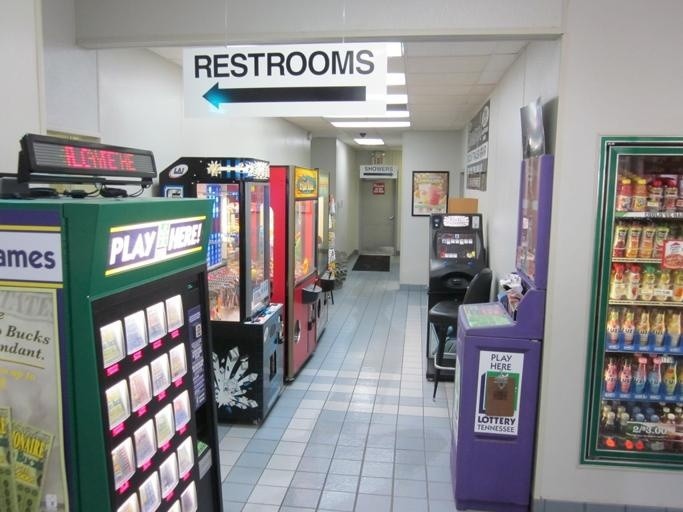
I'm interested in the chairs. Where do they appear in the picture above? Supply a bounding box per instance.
[428,268,492,399]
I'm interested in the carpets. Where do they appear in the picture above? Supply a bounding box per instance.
[352,254,390,271]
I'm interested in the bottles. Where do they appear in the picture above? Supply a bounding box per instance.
[605,306,683,347]
[604,352,683,396]
[615,169,683,213]
[599,401,683,453]
[609,262,683,302]
[612,219,683,259]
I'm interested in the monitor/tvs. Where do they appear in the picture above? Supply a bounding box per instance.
[435,231,477,261]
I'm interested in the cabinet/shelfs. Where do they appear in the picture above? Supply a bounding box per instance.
[579,137,682,469]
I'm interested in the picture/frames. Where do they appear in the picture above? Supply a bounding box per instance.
[412,171,448,216]
